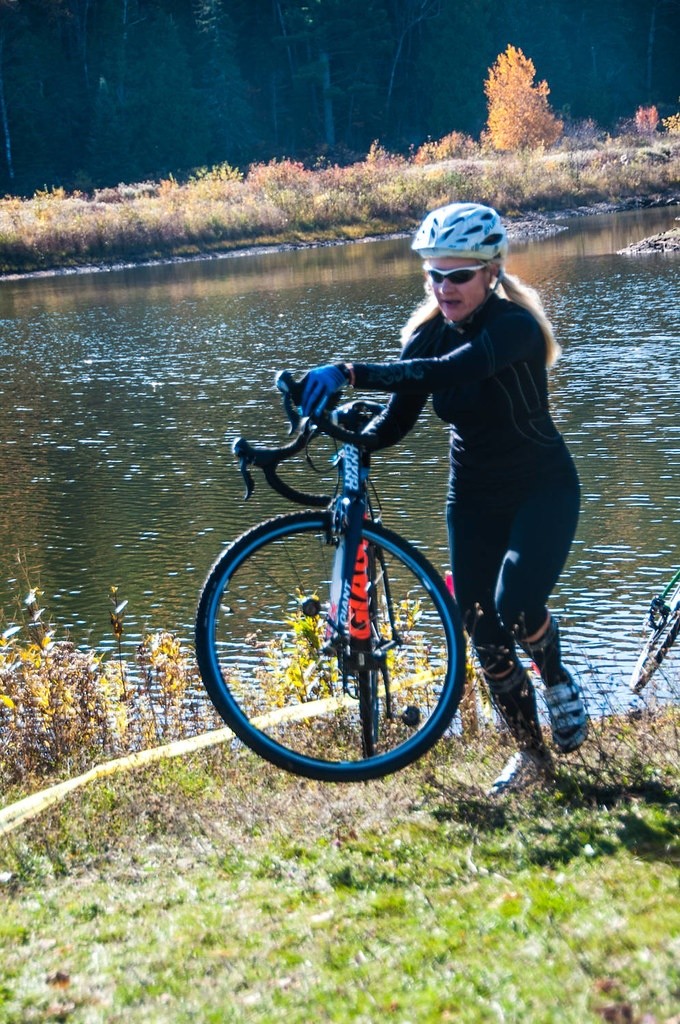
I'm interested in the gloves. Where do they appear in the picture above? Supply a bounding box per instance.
[292,360,349,421]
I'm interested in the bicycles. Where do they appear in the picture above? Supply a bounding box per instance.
[628,569,680,695]
[195,369,467,783]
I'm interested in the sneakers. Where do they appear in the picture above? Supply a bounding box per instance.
[487,746,555,796]
[543,664,587,752]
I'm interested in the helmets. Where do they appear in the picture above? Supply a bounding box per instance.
[410,202,506,260]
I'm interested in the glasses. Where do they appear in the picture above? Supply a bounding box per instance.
[422,259,487,285]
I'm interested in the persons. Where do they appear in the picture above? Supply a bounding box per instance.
[297,204,588,795]
